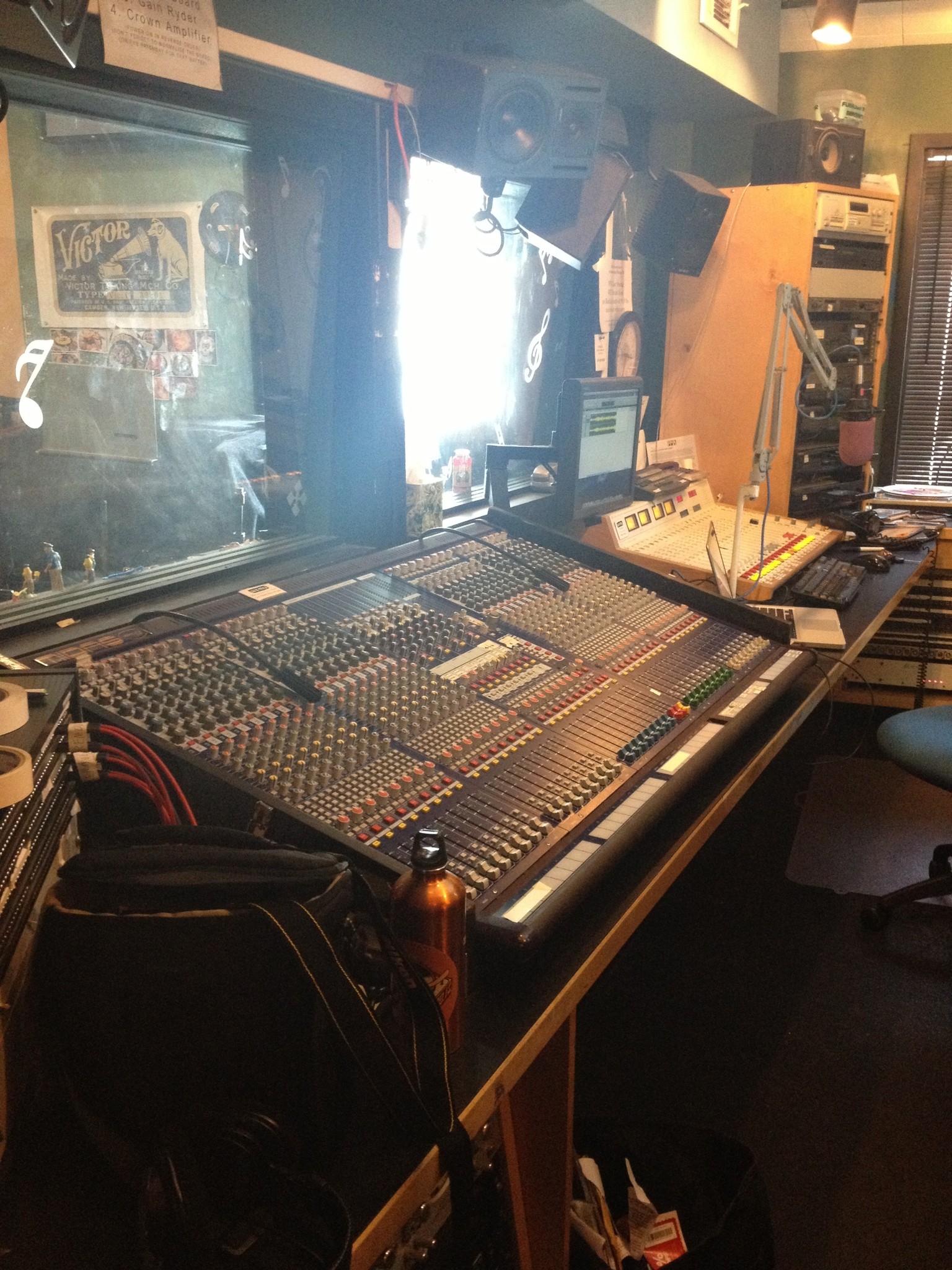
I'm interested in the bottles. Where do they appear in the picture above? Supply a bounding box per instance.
[452,449,472,496]
[390,830,467,1073]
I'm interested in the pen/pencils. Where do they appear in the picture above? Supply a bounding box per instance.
[839,546,884,551]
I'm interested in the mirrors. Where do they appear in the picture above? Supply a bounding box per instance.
[0,68,339,639]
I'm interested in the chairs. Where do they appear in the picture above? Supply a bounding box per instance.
[858,706,952,929]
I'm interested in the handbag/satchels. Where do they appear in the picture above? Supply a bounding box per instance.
[0,825,512,1270]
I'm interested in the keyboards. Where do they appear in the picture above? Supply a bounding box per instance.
[791,555,868,608]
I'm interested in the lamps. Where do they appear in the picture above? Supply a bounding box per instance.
[811,0,858,46]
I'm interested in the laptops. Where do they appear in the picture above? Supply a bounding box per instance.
[705,520,846,651]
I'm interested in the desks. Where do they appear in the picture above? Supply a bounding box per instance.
[860,493,952,514]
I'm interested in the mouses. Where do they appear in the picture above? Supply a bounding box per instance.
[851,554,892,573]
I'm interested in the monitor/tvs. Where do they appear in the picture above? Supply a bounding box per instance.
[554,376,643,520]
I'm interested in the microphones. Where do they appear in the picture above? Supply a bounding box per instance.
[833,363,887,467]
[420,526,572,593]
[130,611,324,704]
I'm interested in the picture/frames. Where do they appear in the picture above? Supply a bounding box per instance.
[41,105,172,143]
[30,201,211,332]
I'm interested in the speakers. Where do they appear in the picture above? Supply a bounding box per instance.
[749,118,866,188]
[630,166,732,278]
[414,49,609,178]
[514,146,639,273]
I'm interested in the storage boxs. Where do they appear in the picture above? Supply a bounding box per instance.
[813,89,866,126]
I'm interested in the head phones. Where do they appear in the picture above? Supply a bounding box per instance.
[137,1103,355,1270]
